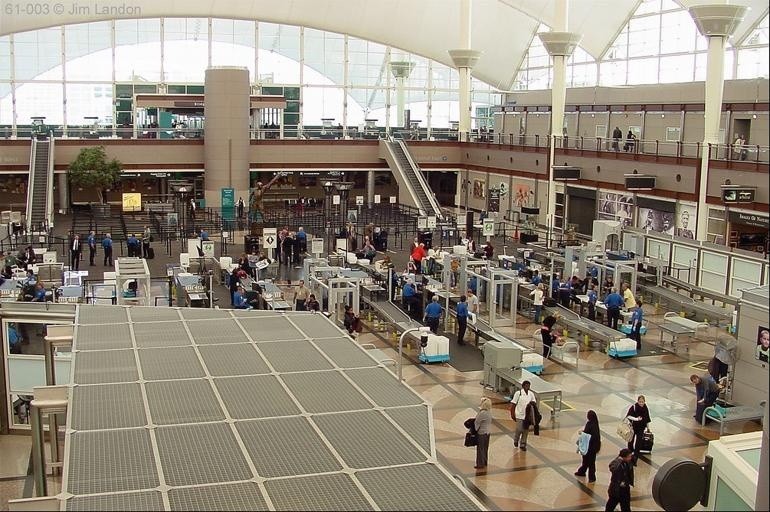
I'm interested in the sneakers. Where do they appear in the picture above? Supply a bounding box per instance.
[514,442,518,447]
[520,445,527,451]
[575,472,585,476]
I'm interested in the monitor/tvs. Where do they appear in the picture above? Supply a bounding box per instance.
[298,176,317,186]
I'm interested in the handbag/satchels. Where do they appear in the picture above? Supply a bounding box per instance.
[464,433,478,446]
[616,417,634,442]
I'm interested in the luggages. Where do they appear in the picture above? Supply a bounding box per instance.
[542,296,557,307]
[695,402,712,425]
[642,431,654,451]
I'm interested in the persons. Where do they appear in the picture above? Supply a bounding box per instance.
[234,197,246,217]
[359,231,495,346]
[678,210,693,239]
[189,199,196,219]
[626,395,651,466]
[612,126,622,151]
[340,227,351,238]
[574,410,600,483]
[229,226,320,311]
[689,374,721,421]
[529,269,643,358]
[344,305,359,341]
[249,171,283,223]
[0,245,45,301]
[510,380,537,451]
[9,322,23,354]
[625,130,637,151]
[201,229,209,240]
[756,330,769,363]
[470,397,494,469]
[734,133,746,160]
[605,448,639,511]
[70,223,151,271]
